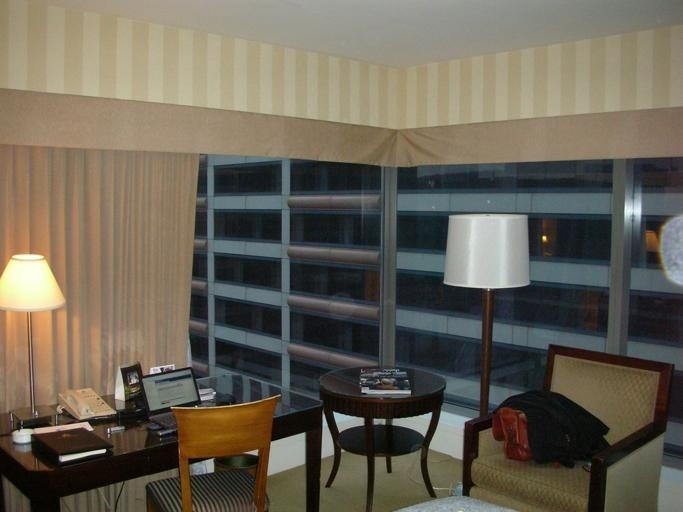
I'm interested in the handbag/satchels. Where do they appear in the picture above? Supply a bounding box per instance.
[492,405,536,464]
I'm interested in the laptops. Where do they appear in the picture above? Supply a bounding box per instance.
[139,367,201,430]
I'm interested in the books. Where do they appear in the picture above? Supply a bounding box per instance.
[31,427,114,466]
[358,368,412,395]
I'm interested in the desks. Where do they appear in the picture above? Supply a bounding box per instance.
[319,367,444,512]
[0,367,324,512]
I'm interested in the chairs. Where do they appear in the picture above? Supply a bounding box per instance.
[462,344,675,512]
[145,394,279,511]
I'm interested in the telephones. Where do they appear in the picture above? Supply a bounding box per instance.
[56,386,117,422]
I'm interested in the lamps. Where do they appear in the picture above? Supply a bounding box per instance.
[445,214,528,415]
[0,254,67,426]
